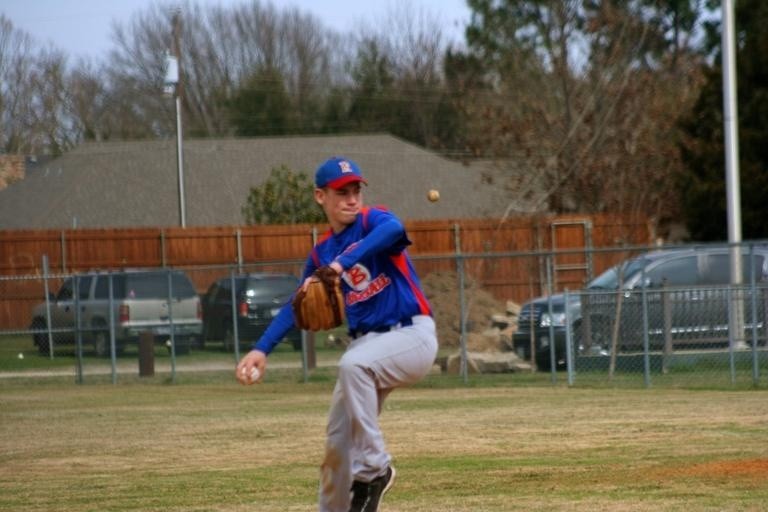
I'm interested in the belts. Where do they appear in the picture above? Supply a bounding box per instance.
[351,317,412,339]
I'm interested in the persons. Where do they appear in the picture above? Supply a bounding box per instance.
[235,158,439,512]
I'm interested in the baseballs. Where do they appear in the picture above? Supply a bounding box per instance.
[242,365,261,384]
[428,190,439,201]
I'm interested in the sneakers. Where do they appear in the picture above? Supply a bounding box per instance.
[348,467,395,512]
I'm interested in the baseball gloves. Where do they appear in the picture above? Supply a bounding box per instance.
[292,266,346,332]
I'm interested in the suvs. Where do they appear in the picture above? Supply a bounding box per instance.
[201,272,304,353]
[29,267,204,357]
[513,242,767,373]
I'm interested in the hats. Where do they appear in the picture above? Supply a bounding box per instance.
[315,157,368,190]
